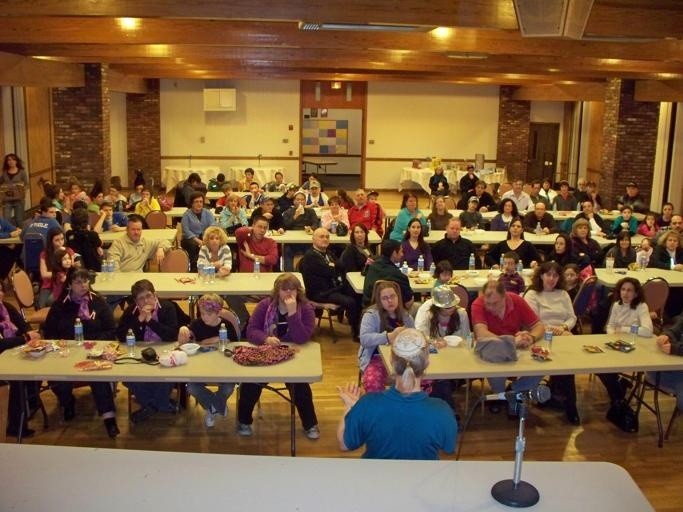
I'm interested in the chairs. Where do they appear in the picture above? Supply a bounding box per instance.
[635,369,683,442]
[637,276,669,335]
[183,306,243,416]
[11,267,52,334]
[290,252,341,340]
[156,248,192,300]
[20,232,46,296]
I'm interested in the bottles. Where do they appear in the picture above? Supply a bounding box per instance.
[427,220,431,236]
[536,222,541,237]
[544,325,553,353]
[331,216,336,234]
[430,263,436,277]
[108,260,113,281]
[577,203,580,212]
[203,263,209,284]
[101,260,107,281]
[402,261,408,276]
[469,253,475,272]
[74,319,83,345]
[119,201,123,212]
[110,256,114,274]
[127,329,135,356]
[418,255,424,274]
[219,323,227,353]
[640,256,646,273]
[197,259,204,280]
[254,258,260,278]
[517,260,523,275]
[631,319,641,346]
[500,254,504,272]
[209,262,215,284]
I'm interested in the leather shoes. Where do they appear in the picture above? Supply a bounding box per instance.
[104,417,119,437]
[63,406,74,420]
[566,408,580,425]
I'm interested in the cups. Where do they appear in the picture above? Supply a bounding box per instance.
[605,257,614,274]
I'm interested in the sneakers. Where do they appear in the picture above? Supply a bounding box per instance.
[205,409,217,427]
[5,426,35,438]
[215,406,228,416]
[304,426,320,439]
[237,422,252,437]
[166,398,178,414]
[131,406,152,423]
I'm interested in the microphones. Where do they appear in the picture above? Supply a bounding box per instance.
[485,386,551,404]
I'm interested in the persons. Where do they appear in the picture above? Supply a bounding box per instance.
[0,216,23,275]
[604,232,636,267]
[427,195,454,231]
[432,218,482,270]
[433,260,453,286]
[573,178,586,202]
[458,180,497,212]
[530,181,550,209]
[472,280,544,417]
[458,196,484,230]
[553,180,575,211]
[358,280,415,391]
[524,262,581,425]
[66,208,104,273]
[523,202,556,234]
[337,328,457,459]
[485,183,501,204]
[485,217,543,268]
[36,227,77,309]
[45,184,62,209]
[277,183,298,211]
[239,169,260,192]
[227,215,278,328]
[571,218,601,258]
[88,191,104,214]
[366,189,379,203]
[180,294,239,427]
[576,181,602,213]
[348,189,382,236]
[362,239,421,318]
[216,185,243,209]
[182,173,201,207]
[321,196,350,230]
[302,172,324,192]
[1,282,42,438]
[545,233,582,266]
[618,181,643,212]
[64,182,91,205]
[108,217,170,309]
[390,192,426,241]
[43,267,120,439]
[416,287,470,405]
[32,197,63,224]
[597,277,653,403]
[238,273,320,439]
[104,184,127,204]
[307,180,329,206]
[637,238,655,268]
[490,198,519,231]
[538,179,557,202]
[73,200,88,212]
[250,195,286,234]
[94,202,127,234]
[460,165,479,193]
[638,211,660,237]
[116,279,180,423]
[576,200,608,236]
[50,249,71,299]
[646,230,683,272]
[304,227,362,342]
[282,192,318,232]
[656,316,683,357]
[342,223,373,311]
[19,202,62,276]
[656,215,683,247]
[0,153,27,228]
[659,202,674,226]
[429,167,454,196]
[563,264,580,298]
[611,206,637,237]
[398,218,432,270]
[129,184,144,207]
[182,192,215,270]
[335,189,355,209]
[240,183,265,208]
[135,187,161,218]
[270,173,286,192]
[501,178,535,214]
[220,195,249,233]
[197,226,232,273]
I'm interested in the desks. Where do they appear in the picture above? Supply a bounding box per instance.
[225,227,383,270]
[202,190,288,205]
[592,232,652,252]
[401,167,452,202]
[592,266,681,320]
[378,332,681,448]
[0,340,321,456]
[135,204,188,230]
[547,207,647,227]
[455,164,493,192]
[415,227,560,269]
[381,206,502,237]
[344,270,540,309]
[87,269,306,326]
[304,158,338,183]
[0,229,182,273]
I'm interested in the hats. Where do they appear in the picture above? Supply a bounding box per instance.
[309,180,320,189]
[432,285,460,308]
[261,194,273,202]
[468,196,479,203]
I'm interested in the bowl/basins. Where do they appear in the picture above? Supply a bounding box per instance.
[467,270,480,277]
[28,350,47,358]
[419,274,431,278]
[443,336,462,347]
[313,206,320,213]
[489,270,502,275]
[180,343,201,354]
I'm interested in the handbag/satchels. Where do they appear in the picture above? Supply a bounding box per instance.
[476,335,518,362]
